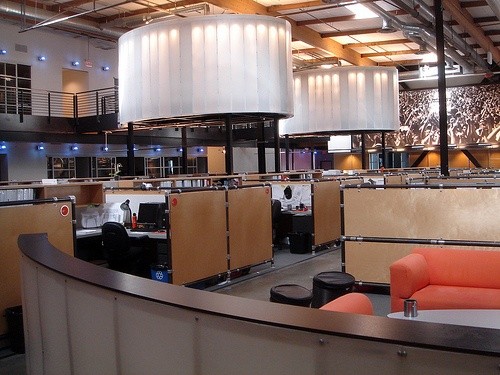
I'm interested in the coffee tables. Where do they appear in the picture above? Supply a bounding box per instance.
[387,309,500,330]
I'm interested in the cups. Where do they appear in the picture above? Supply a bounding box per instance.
[403,299,419,317]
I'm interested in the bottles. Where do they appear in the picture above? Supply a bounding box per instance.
[132,212,137,229]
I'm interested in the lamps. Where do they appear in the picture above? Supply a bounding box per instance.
[120,199,132,229]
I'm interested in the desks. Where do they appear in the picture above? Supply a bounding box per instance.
[292,215,313,233]
[76,228,171,240]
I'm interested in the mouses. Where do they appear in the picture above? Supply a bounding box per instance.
[153,229,158,232]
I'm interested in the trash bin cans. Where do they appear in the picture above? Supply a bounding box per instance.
[150,256,171,283]
[288,231,312,254]
[5,305,25,354]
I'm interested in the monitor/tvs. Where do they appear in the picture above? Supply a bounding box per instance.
[137,202,160,229]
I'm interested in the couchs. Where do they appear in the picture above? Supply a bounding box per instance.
[319,292,374,316]
[390,248,500,313]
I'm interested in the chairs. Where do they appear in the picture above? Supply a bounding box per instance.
[271,200,296,250]
[102,222,154,278]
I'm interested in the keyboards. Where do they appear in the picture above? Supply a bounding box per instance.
[130,228,154,232]
